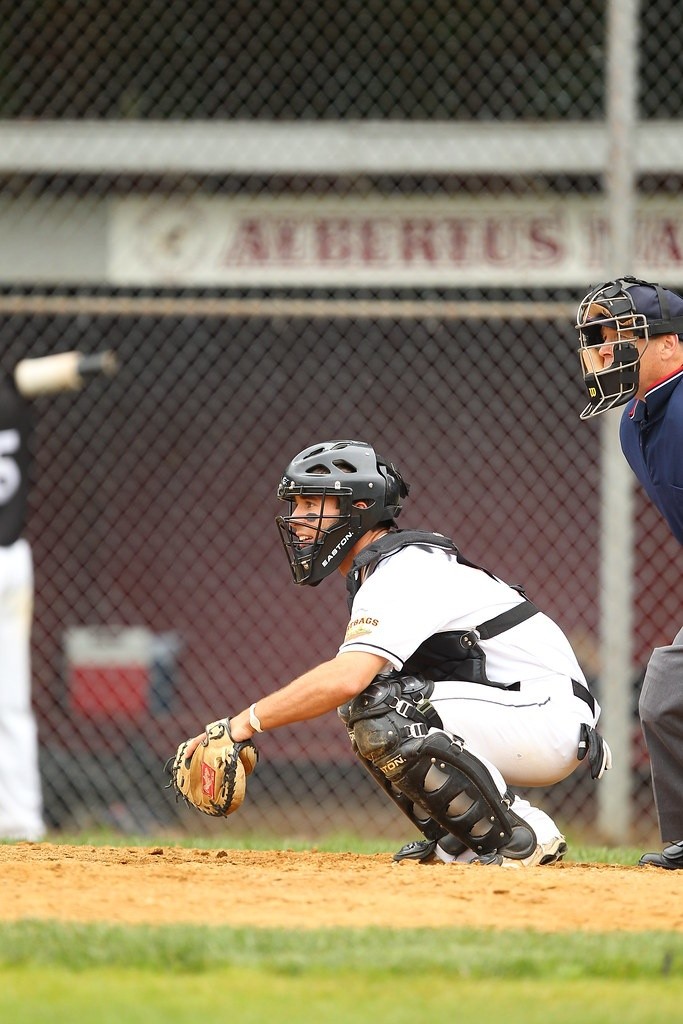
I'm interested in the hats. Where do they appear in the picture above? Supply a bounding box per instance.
[591,285,682,337]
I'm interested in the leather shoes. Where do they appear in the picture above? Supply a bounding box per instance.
[637,839,683,872]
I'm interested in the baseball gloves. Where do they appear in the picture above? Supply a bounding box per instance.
[164,718,261,816]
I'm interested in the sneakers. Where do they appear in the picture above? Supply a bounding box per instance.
[394,806,568,868]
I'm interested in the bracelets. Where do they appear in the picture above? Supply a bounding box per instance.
[248,702,265,734]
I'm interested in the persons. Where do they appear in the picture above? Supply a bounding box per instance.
[576,277,683,871]
[163,440,611,868]
[0,392,50,841]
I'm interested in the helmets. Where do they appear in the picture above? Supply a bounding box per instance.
[275,439,411,588]
